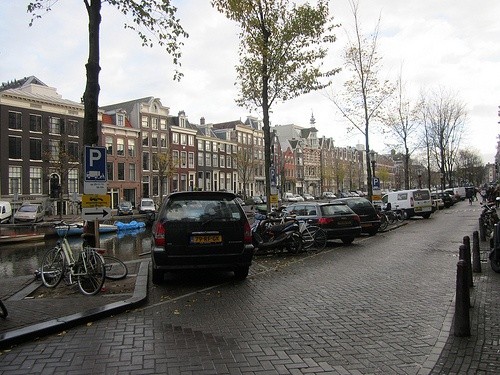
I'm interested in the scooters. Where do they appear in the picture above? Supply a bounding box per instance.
[480,196,500,273]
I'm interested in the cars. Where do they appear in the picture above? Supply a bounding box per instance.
[116,201,134,216]
[332,197,382,237]
[237,197,245,206]
[278,192,315,203]
[250,195,267,205]
[13,204,45,224]
[321,190,368,199]
[138,198,155,214]
[430,186,472,214]
[284,201,361,249]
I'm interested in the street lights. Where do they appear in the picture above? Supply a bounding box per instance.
[440,173,444,190]
[368,149,377,177]
[417,169,422,189]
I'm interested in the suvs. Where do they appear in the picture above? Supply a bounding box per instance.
[146,191,255,285]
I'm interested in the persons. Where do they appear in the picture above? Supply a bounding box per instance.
[480,186,500,207]
[466,189,474,202]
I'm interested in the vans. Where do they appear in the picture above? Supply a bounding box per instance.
[0,200,12,223]
[380,188,432,220]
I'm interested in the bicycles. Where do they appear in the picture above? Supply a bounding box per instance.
[32,220,129,296]
[250,204,327,257]
[373,203,407,232]
